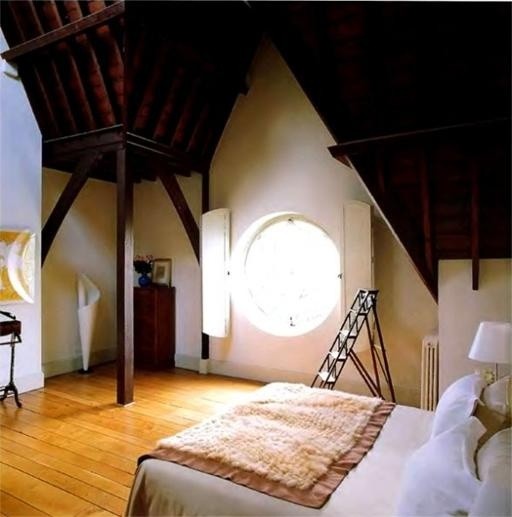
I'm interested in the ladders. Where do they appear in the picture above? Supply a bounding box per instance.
[311,289,396,404]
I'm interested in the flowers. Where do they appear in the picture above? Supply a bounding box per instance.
[134,255,153,274]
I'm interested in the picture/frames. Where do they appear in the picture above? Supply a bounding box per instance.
[0,229,36,305]
[152,258,172,287]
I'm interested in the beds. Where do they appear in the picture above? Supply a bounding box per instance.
[124,381,435,517]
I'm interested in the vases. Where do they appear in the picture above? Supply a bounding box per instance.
[139,273,151,287]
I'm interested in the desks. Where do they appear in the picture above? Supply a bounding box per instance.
[0,311,22,408]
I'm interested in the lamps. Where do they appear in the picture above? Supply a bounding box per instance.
[468,321,512,381]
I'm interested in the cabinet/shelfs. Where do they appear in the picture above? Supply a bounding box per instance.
[133,287,175,371]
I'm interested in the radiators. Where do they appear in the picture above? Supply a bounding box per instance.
[419,335,439,410]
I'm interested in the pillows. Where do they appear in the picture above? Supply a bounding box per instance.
[474,375,512,445]
[430,374,485,441]
[467,427,512,517]
[396,416,483,517]
